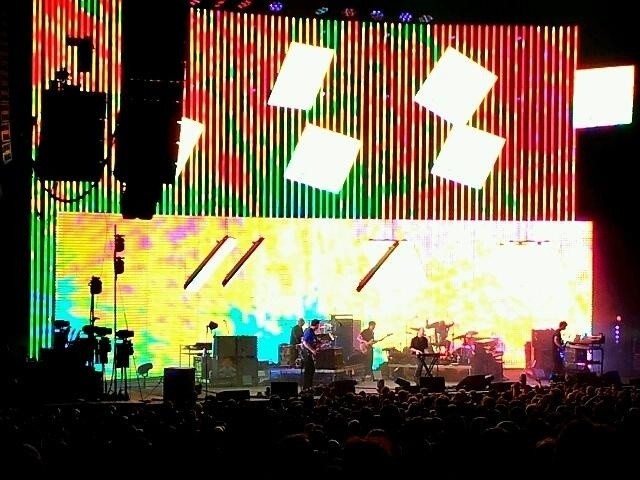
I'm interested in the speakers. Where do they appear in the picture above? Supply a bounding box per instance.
[490,381,518,391]
[602,370,621,385]
[328,380,355,393]
[216,390,250,400]
[164,368,195,405]
[271,382,298,398]
[418,376,445,393]
[456,374,487,391]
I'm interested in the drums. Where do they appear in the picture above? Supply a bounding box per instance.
[295,359,302,367]
[296,343,306,350]
[281,345,298,367]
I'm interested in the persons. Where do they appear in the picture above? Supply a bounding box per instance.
[289,319,306,364]
[0,373,638,478]
[552,321,569,373]
[299,320,321,388]
[410,327,428,376]
[357,321,377,382]
[426,320,455,354]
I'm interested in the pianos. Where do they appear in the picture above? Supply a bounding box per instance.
[416,353,446,356]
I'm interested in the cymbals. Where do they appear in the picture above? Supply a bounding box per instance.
[410,327,426,332]
[323,320,343,327]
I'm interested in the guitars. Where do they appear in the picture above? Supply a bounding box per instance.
[359,333,393,356]
